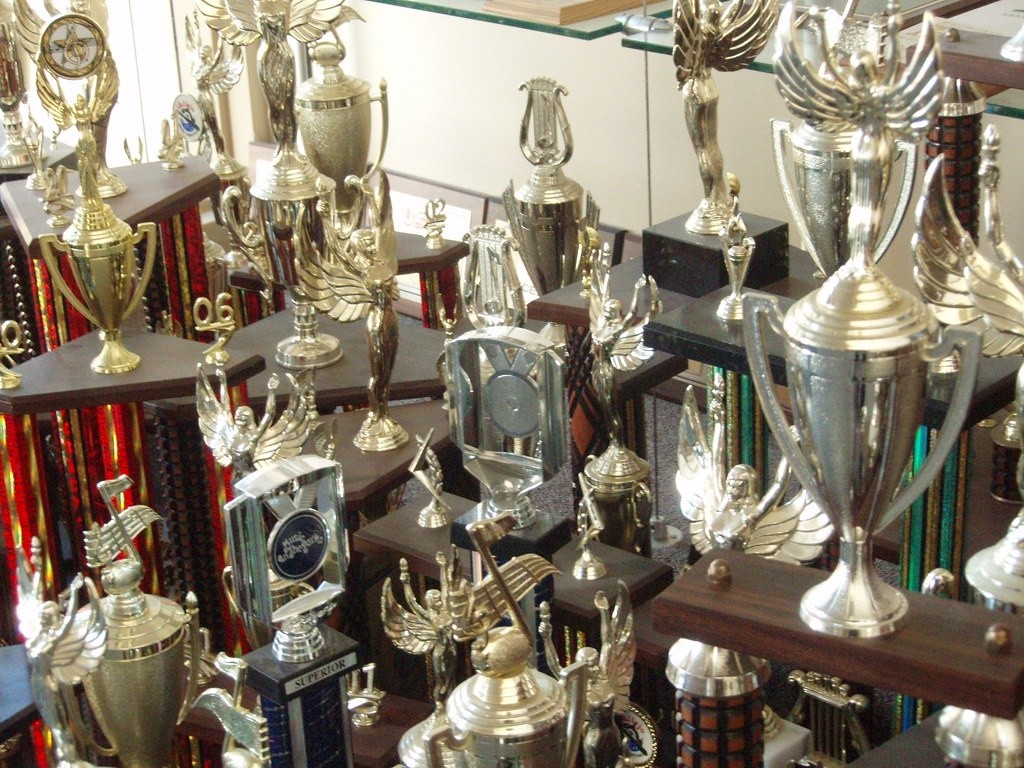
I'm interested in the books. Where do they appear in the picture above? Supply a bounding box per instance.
[896,0,1024,91]
[0,644,38,732]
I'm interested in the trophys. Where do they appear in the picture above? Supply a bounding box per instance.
[0,0,1023,768]
